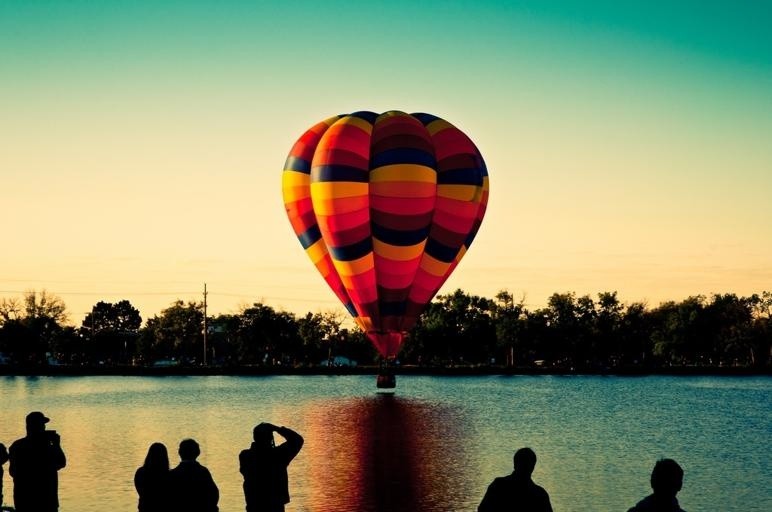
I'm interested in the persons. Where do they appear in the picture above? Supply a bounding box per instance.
[161,436,220,512]
[6,410,66,512]
[476,447,554,511]
[625,457,688,511]
[133,442,171,511]
[238,421,304,511]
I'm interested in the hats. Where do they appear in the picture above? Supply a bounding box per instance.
[26,412,49,424]
